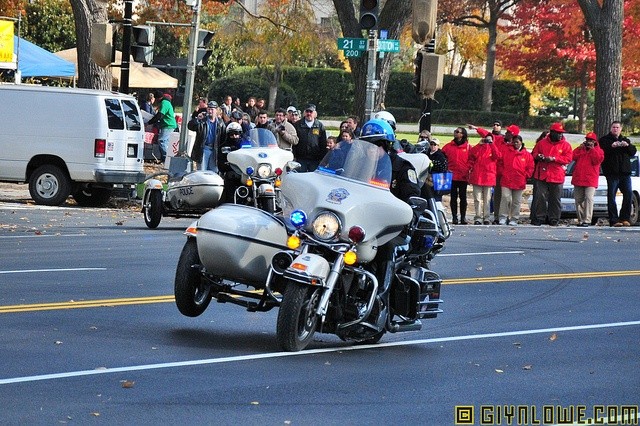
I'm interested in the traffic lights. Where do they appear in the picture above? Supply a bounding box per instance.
[129,46,154,63]
[196,50,212,67]
[194,26,215,48]
[133,25,153,44]
[359,13,376,31]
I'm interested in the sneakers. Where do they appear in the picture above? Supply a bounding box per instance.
[498,217,508,224]
[510,221,519,224]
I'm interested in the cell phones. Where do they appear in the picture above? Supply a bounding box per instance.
[481,137,492,144]
[202,112,210,117]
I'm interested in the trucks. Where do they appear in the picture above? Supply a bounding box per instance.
[0,83,145,206]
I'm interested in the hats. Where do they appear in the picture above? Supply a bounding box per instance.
[585,132,597,140]
[207,100,219,108]
[506,125,520,136]
[430,138,440,145]
[287,106,296,112]
[306,104,316,111]
[550,123,566,132]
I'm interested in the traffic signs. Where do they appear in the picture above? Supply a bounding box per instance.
[337,38,366,51]
[377,40,399,53]
[344,51,361,58]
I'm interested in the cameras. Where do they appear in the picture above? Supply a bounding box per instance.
[618,142,624,146]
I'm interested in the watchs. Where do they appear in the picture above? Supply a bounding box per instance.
[553,157,555,161]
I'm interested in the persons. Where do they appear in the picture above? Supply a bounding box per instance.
[268,108,299,152]
[231,96,244,119]
[418,130,431,198]
[466,124,520,225]
[220,95,233,127]
[442,127,475,224]
[144,94,177,165]
[426,138,448,201]
[244,113,252,125]
[530,131,553,220]
[255,98,266,110]
[374,111,397,139]
[347,115,361,138]
[187,101,227,173]
[243,97,260,123]
[253,110,279,147]
[326,136,337,150]
[571,132,604,227]
[532,122,572,226]
[598,121,637,227]
[293,110,302,123]
[343,130,353,140]
[141,93,157,115]
[287,106,301,125]
[191,97,208,119]
[328,119,421,309]
[490,120,502,214]
[471,133,502,225]
[217,122,282,213]
[293,103,327,172]
[497,135,534,226]
[336,121,348,143]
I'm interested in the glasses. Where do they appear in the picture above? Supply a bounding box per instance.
[294,114,298,116]
[454,130,461,133]
[431,143,436,146]
[259,117,266,119]
[494,124,501,126]
[276,114,283,117]
[420,136,427,138]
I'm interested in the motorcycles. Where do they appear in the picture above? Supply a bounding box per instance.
[141,127,301,230]
[173,138,455,353]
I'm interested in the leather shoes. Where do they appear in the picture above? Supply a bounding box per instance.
[462,221,469,224]
[581,223,590,226]
[493,221,496,224]
[453,222,458,225]
[475,221,480,225]
[624,222,631,225]
[550,219,559,224]
[484,221,489,224]
[613,222,624,226]
[577,223,583,226]
[534,220,541,225]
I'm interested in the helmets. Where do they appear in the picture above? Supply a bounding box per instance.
[374,110,396,127]
[226,123,243,132]
[360,119,396,140]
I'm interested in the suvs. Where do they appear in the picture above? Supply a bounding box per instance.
[543,152,640,226]
